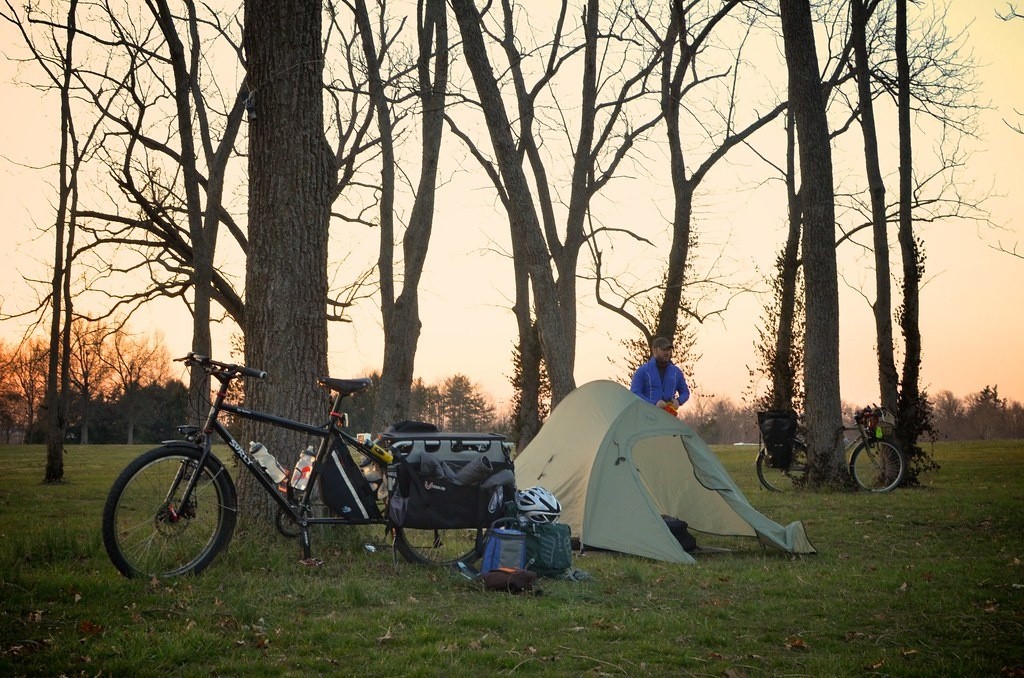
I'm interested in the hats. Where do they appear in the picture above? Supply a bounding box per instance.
[653,337,674,350]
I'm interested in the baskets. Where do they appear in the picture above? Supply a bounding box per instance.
[868,425,893,438]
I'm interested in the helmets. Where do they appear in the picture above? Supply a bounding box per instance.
[516,486,562,524]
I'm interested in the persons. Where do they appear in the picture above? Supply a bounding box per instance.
[631,338,690,417]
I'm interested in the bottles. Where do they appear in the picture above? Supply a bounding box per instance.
[357,459,388,500]
[249,441,286,483]
[289,445,317,491]
[362,438,393,463]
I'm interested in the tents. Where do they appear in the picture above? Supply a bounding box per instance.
[514,380,819,565]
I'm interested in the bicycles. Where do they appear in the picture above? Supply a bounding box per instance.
[755,403,906,494]
[100,351,500,587]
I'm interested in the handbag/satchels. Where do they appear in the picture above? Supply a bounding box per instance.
[522,521,571,578]
[388,460,515,528]
[757,410,795,468]
